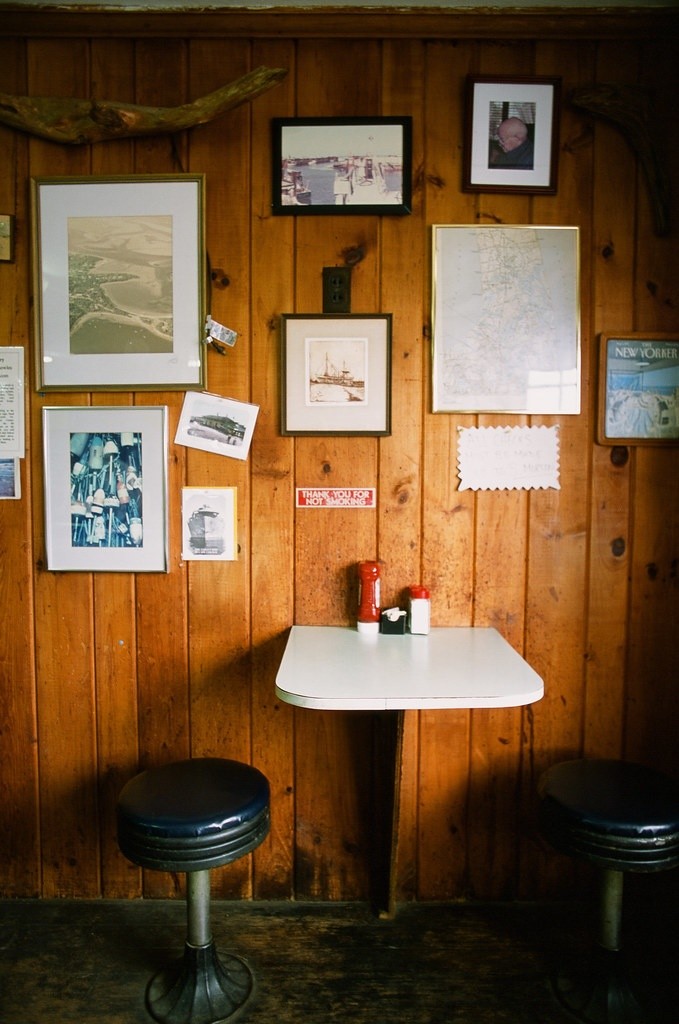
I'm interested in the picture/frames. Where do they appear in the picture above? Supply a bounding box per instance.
[41,404,171,574]
[30,171,207,392]
[597,330,679,447]
[279,314,392,436]
[272,116,412,216]
[461,71,561,197]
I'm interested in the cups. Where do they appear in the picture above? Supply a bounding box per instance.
[357,560,382,635]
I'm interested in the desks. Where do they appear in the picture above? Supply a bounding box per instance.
[276,625,545,918]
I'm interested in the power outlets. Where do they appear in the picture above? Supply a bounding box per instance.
[321,265,351,313]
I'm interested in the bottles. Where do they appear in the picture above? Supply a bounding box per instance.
[407,585,431,635]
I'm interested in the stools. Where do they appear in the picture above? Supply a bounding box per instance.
[114,756,271,1024]
[536,758,679,1024]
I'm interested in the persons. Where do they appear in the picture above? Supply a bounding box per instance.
[493,117,533,170]
